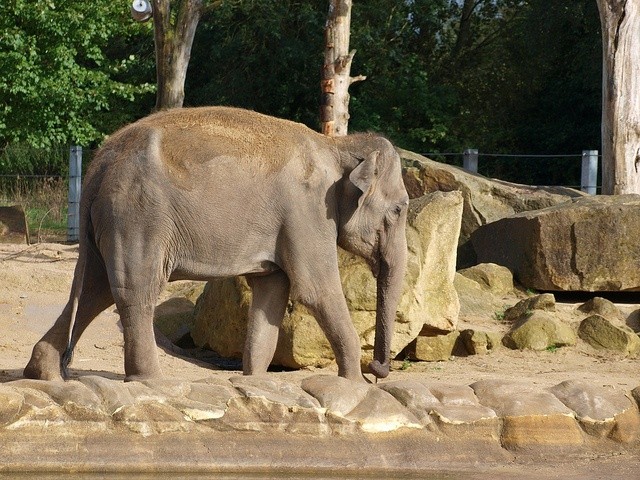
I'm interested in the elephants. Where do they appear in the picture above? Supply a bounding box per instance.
[22,106,410,385]
